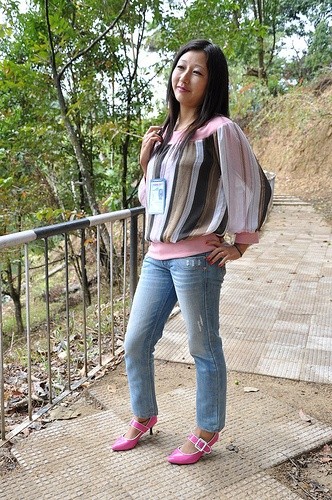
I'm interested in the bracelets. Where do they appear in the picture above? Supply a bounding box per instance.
[234,243,243,259]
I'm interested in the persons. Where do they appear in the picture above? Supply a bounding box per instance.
[112,40,273,464]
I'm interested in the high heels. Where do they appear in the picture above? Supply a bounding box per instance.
[112,415,157,451]
[167,431,219,464]
